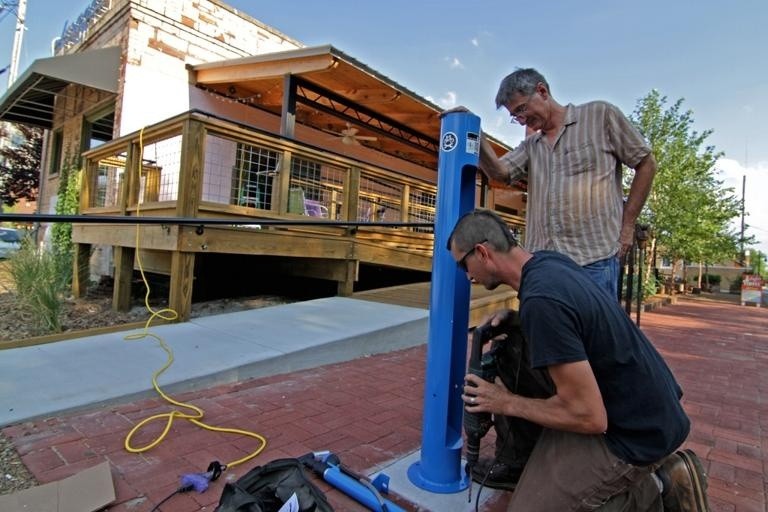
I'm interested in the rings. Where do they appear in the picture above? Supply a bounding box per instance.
[470,397,475,405]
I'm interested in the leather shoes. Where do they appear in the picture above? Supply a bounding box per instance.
[465,457,521,490]
[655,447,711,512]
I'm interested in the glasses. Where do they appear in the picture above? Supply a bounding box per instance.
[510,87,537,125]
[456,238,489,273]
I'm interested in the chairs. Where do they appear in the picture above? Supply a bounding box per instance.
[291,188,329,217]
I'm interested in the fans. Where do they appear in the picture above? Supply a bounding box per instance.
[321,123,378,145]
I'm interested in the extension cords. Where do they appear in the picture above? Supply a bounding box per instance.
[181,470,211,493]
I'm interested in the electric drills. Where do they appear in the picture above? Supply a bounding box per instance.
[463,319,515,503]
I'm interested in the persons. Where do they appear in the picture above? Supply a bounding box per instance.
[446,210,712,510]
[436,66,660,304]
